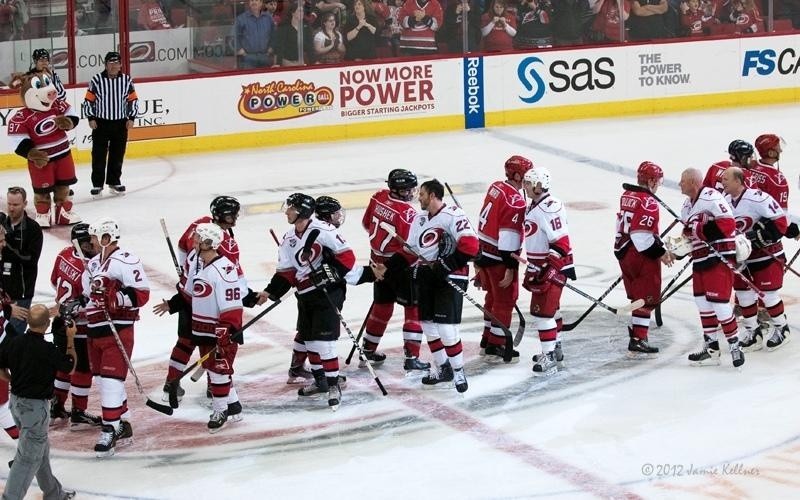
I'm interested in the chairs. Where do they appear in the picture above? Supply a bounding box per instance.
[19,16,51,40]
[703,12,799,40]
[122,0,239,75]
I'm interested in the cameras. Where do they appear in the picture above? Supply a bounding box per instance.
[61,308,73,329]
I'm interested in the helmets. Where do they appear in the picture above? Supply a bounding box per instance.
[638,134,787,186]
[106,52,121,63]
[72,220,120,247]
[281,193,345,225]
[385,156,551,201]
[188,196,239,250]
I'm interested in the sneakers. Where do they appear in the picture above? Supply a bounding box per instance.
[91,185,125,195]
[688,304,791,368]
[479,334,562,373]
[50,404,132,453]
[359,348,467,394]
[64,490,75,500]
[627,325,658,354]
[289,364,346,408]
[163,376,241,430]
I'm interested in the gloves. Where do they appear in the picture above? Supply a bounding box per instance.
[89,286,119,314]
[215,322,233,354]
[529,254,565,285]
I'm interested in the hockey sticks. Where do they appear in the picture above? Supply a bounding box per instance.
[562,218,679,332]
[168,286,298,409]
[619,165,800,333]
[302,228,389,396]
[270,228,280,247]
[445,182,527,348]
[66,203,392,418]
[345,282,381,364]
[381,222,514,364]
[161,217,206,382]
[72,238,174,416]
[511,252,645,316]
[377,181,651,364]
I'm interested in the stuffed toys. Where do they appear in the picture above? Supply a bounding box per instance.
[7,68,81,229]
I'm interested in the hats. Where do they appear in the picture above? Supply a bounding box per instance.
[33,49,49,64]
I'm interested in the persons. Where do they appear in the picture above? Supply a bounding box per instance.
[83,50,139,199]
[0,303,82,499]
[1,128,796,458]
[1,0,799,67]
[27,48,67,109]
